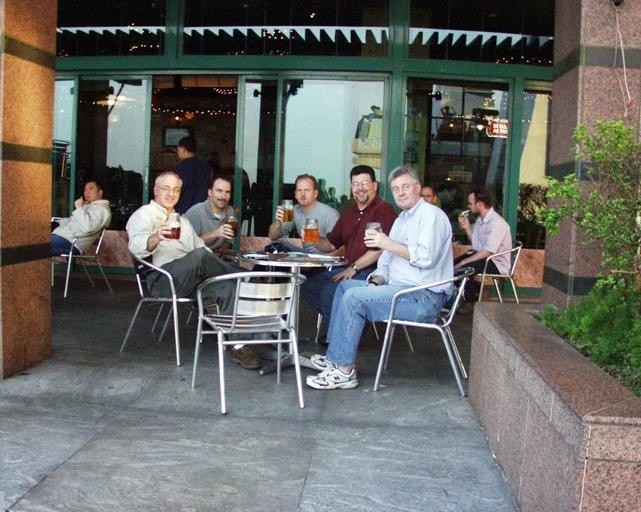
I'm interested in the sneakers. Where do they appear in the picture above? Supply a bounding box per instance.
[456,301,475,314]
[229,344,264,371]
[304,360,360,390]
[308,352,328,368]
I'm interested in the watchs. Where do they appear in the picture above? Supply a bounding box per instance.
[351,262,361,274]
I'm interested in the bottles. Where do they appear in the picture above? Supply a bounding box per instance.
[366,222,383,251]
[227,215,239,237]
[281,199,295,222]
[165,212,181,239]
[304,218,320,245]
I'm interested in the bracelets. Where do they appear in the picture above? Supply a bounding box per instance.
[369,279,378,286]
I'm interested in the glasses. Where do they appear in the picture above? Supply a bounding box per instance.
[155,185,181,192]
[353,180,373,187]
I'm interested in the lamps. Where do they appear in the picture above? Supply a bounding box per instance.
[254,89,261,97]
[96,87,113,99]
[424,89,441,100]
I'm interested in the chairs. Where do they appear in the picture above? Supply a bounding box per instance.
[50,217,116,298]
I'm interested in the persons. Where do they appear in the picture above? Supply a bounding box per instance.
[50,178,114,258]
[126,169,271,369]
[302,164,396,346]
[421,184,437,204]
[305,167,455,390]
[182,176,241,251]
[452,190,514,314]
[175,136,212,219]
[270,174,340,279]
